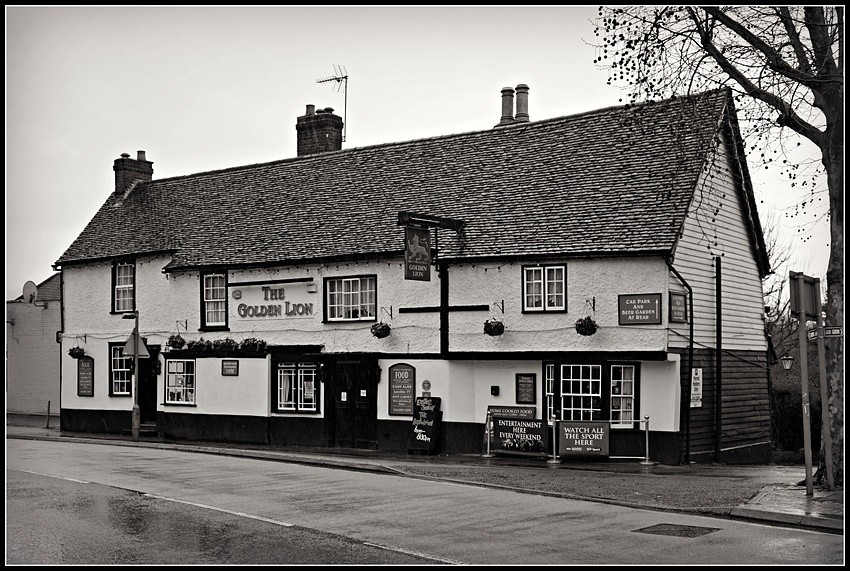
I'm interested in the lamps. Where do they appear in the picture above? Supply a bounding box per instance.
[781,352,794,376]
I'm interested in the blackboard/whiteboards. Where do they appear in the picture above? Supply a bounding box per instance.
[408,397,442,450]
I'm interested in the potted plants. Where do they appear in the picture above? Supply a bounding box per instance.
[575,316,599,336]
[484,319,504,336]
[68,346,85,359]
[168,335,185,349]
[371,321,391,339]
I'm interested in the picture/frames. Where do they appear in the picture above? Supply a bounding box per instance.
[516,373,536,404]
[222,360,239,376]
